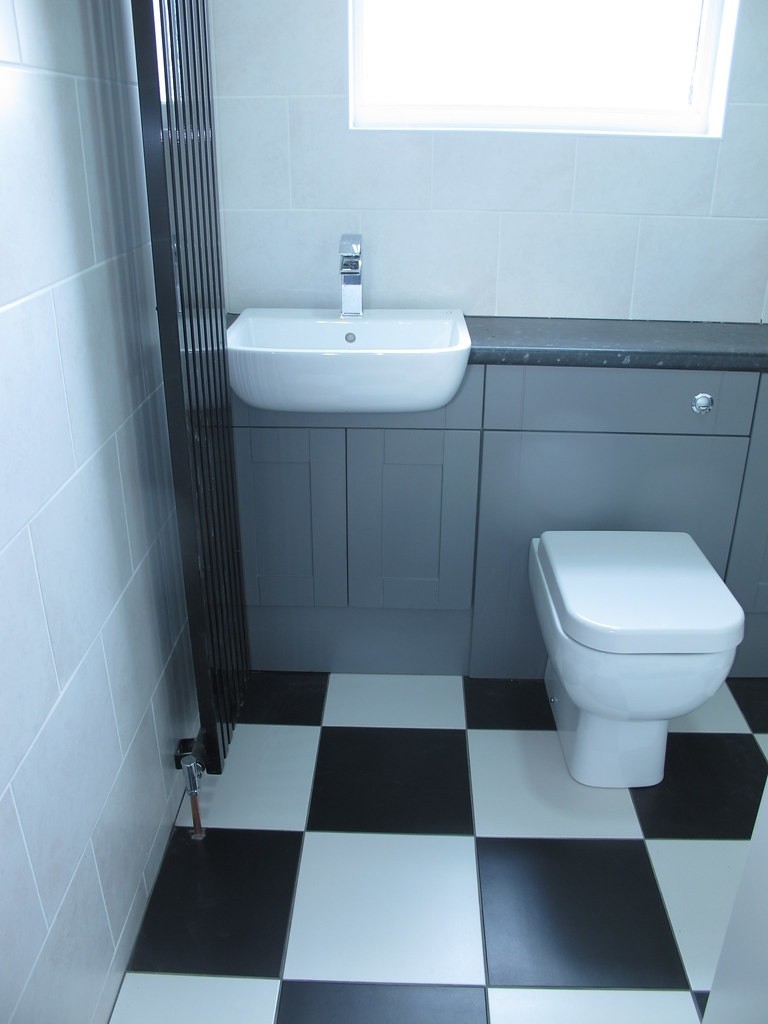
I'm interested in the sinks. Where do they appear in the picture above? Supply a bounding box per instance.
[226,307,472,410]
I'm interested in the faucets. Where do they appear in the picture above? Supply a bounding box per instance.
[339,233,363,315]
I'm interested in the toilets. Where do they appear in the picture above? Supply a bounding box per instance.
[529,532,747,787]
